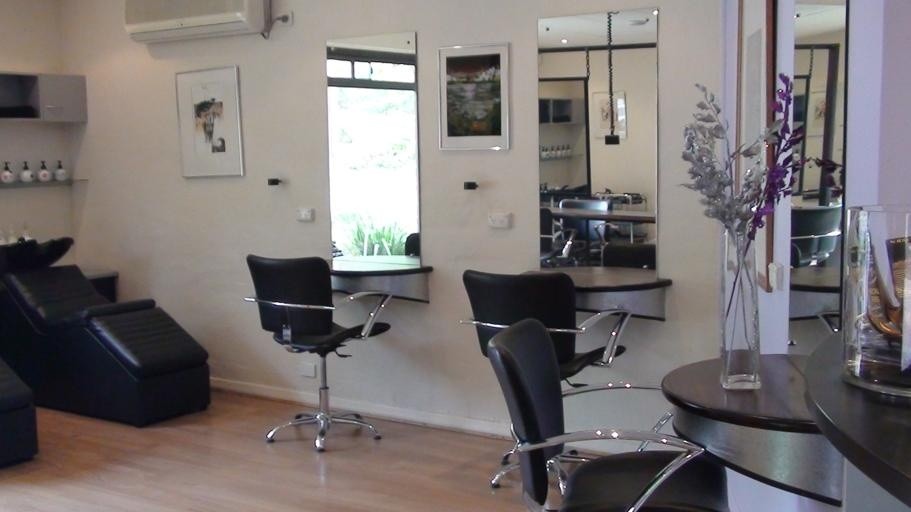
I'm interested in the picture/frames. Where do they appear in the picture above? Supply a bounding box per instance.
[174,64,246,178]
[437,42,510,150]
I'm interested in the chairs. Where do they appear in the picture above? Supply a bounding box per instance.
[488,318,730,512]
[243,254,395,452]
[459,269,632,498]
[541,199,610,267]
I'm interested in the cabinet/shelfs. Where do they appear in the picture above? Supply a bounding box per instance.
[0,72,88,123]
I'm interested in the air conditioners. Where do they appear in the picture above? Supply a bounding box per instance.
[118,1,294,40]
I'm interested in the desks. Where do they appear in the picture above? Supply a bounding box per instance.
[804,314,911,512]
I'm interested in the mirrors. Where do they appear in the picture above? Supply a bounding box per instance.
[536,8,657,278]
[790,0,849,360]
[325,31,422,265]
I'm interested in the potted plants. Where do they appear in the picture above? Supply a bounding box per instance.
[682,73,812,389]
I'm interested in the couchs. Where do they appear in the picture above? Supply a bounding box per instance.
[1,237,212,430]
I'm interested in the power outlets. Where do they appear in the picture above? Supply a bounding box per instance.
[296,207,316,222]
[487,212,512,229]
